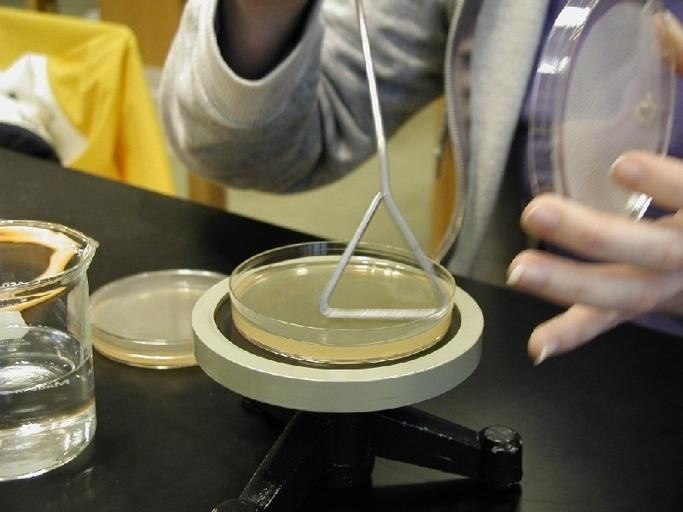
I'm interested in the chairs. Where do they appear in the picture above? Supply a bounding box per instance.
[0,8,174,197]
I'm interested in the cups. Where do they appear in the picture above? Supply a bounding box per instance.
[1,218,99,484]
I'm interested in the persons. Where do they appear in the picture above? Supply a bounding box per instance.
[153,0,682,371]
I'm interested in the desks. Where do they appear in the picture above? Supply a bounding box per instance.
[0,147,683,512]
